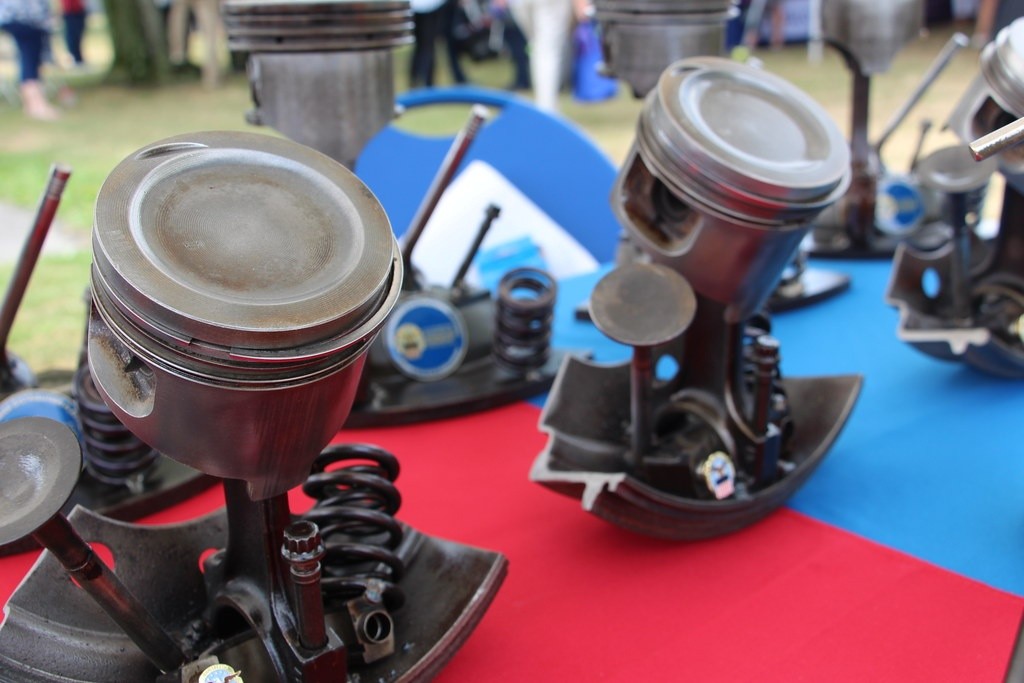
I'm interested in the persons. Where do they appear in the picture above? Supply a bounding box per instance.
[408,0,465,89]
[489,0,530,94]
[509,1,595,114]
[924,0,1024,48]
[59,0,86,63]
[0,0,58,96]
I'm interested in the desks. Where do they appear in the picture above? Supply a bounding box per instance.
[2,254,1024,683]
